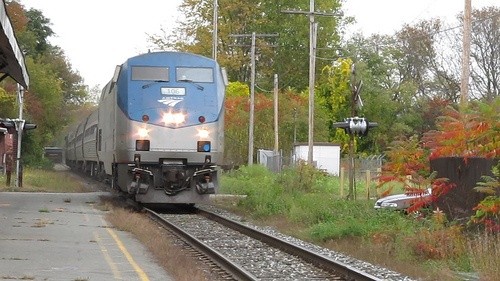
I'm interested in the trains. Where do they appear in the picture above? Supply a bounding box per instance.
[63,49,224,208]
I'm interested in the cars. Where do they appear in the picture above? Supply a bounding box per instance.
[374,187,433,212]
